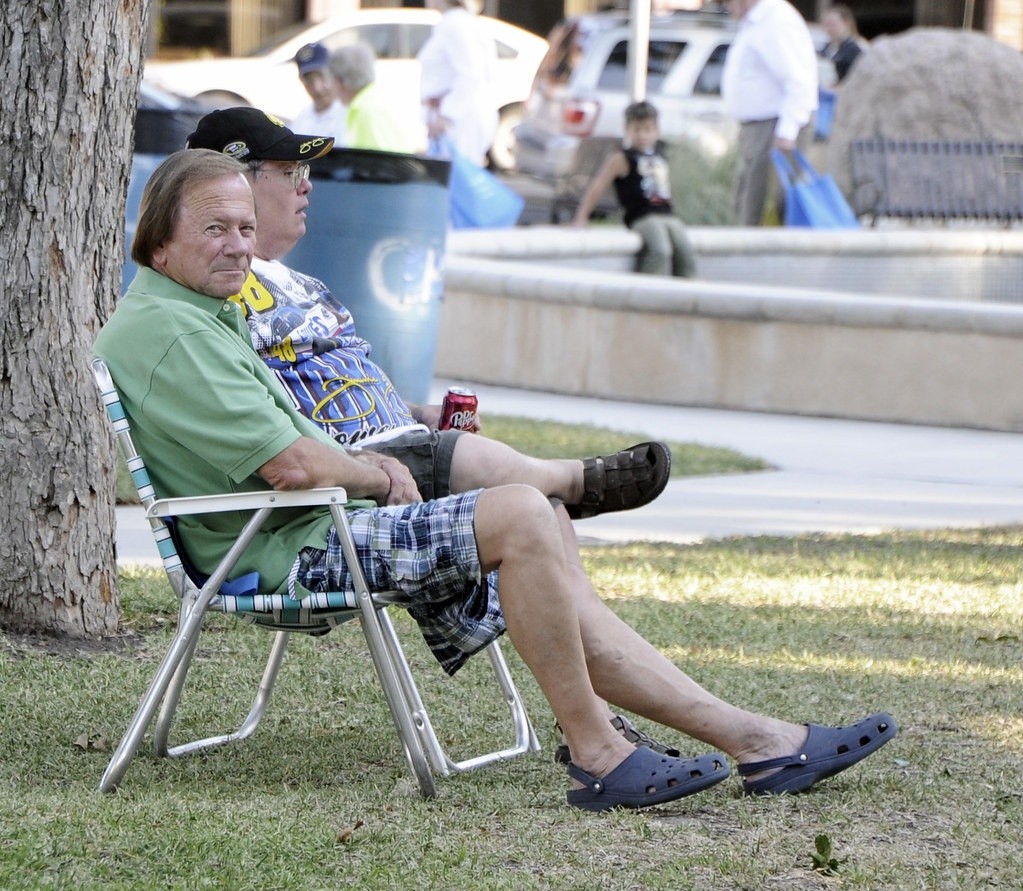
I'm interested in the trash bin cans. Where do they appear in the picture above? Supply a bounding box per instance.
[120,109,452,406]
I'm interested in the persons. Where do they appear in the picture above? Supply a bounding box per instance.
[716,0,818,227]
[416,0,499,171]
[295,42,407,151]
[95,148,897,812]
[187,106,682,763]
[574,100,696,279]
[819,0,865,83]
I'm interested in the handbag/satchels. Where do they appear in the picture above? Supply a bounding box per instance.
[423,130,525,233]
[814,85,836,140]
[769,144,858,230]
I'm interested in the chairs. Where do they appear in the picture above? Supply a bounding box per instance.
[98,357,540,798]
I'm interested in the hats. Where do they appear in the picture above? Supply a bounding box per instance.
[293,43,330,77]
[183,107,335,164]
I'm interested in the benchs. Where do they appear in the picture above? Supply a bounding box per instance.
[847,141,1023,231]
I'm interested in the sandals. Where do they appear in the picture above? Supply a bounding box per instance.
[737,712,897,799]
[555,715,686,767]
[562,440,671,519]
[565,744,728,811]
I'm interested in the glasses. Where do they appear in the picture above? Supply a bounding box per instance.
[244,163,310,190]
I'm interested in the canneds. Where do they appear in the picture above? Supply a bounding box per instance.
[438,386,478,434]
